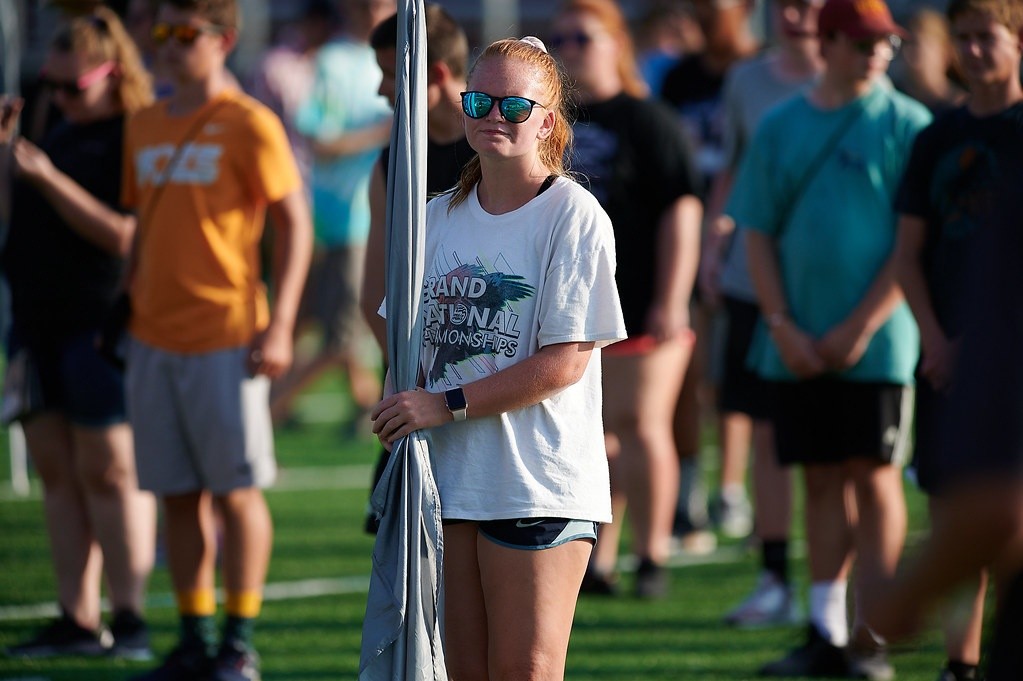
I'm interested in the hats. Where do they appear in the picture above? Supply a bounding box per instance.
[818,0,911,42]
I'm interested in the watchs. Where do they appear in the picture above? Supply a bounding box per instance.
[444,387,468,424]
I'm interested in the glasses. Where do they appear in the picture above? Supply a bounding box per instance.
[548,28,608,50]
[42,55,113,99]
[845,35,897,59]
[460,91,547,124]
[150,21,228,46]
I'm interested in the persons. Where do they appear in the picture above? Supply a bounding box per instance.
[0,5,156,661]
[891,0,1023,681]
[534,0,704,598]
[734,0,929,679]
[122,0,315,681]
[707,0,893,540]
[633,0,705,97]
[359,10,470,364]
[887,7,966,120]
[656,0,748,540]
[372,37,629,681]
[254,0,395,444]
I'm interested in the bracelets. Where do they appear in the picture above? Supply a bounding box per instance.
[769,312,790,326]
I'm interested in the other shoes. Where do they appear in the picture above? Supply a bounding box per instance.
[712,480,753,542]
[805,582,849,649]
[77,605,153,661]
[752,622,897,681]
[137,638,214,681]
[9,611,104,656]
[670,480,711,558]
[723,580,797,627]
[630,555,666,600]
[209,643,259,681]
[931,658,980,681]
[577,564,618,598]
